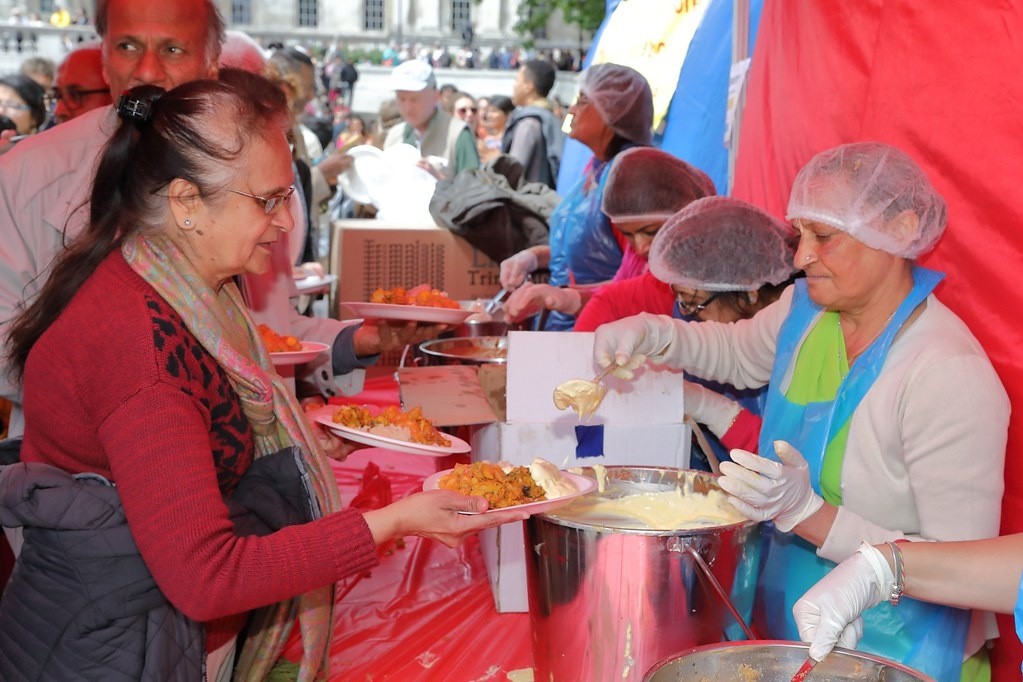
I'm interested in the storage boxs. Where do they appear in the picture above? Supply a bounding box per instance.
[395,326,695,612]
[334,219,506,323]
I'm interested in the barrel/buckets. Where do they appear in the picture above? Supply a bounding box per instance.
[521,464,762,682]
[643,640,943,681]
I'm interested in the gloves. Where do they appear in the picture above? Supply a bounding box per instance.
[499,250,538,292]
[502,281,582,323]
[684,380,743,439]
[592,311,675,380]
[718,440,824,533]
[792,540,897,663]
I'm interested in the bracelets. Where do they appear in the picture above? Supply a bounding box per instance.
[885,541,905,605]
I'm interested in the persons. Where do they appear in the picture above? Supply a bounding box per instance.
[591,141,1009,682]
[0,0,804,682]
[792,531,1022,661]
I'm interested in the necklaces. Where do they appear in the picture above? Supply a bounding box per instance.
[848,310,897,364]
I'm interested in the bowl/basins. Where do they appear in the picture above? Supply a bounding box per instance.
[419,335,508,367]
[440,300,517,335]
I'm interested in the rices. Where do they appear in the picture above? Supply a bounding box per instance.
[350,422,412,441]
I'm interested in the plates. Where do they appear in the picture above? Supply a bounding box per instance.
[338,145,382,203]
[313,414,472,457]
[271,342,330,366]
[340,301,481,325]
[294,274,338,296]
[422,468,597,516]
[382,143,422,183]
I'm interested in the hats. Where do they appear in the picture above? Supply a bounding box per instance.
[387,59,436,92]
[0,73,46,130]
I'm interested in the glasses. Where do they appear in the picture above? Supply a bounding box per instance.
[676,291,726,321]
[150,175,295,214]
[0,100,31,116]
[457,106,478,115]
[42,88,110,112]
